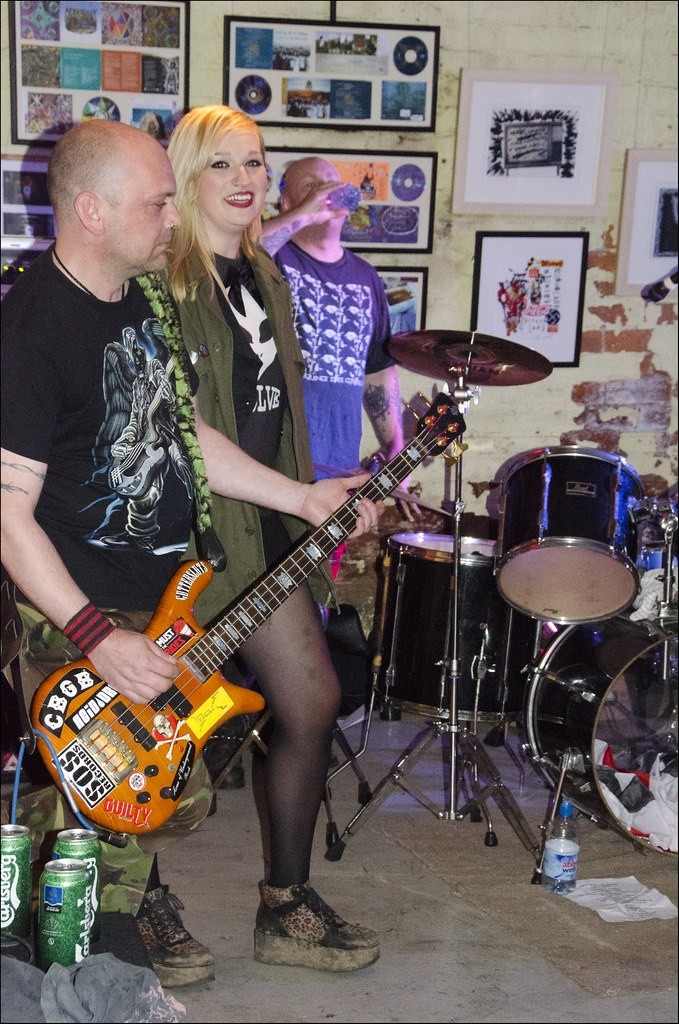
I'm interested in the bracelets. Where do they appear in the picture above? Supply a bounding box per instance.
[63,602,115,655]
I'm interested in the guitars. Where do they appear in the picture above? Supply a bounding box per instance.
[29,389,470,831]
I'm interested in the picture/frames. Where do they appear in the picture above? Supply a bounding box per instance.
[375,266,428,330]
[615,148,678,301]
[452,67,618,218]
[7,1,191,147]
[261,146,438,255]
[471,231,590,367]
[222,15,440,132]
[0,152,56,298]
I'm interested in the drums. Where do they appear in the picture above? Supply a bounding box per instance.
[368,532,535,723]
[489,444,646,625]
[527,610,679,858]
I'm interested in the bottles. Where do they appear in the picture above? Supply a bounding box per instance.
[328,185,360,211]
[541,798,583,894]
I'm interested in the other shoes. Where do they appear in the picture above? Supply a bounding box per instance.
[204,721,248,790]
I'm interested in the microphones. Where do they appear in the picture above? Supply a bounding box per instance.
[650,272,678,302]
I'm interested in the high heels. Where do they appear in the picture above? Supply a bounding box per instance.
[253,879,382,973]
[134,885,215,986]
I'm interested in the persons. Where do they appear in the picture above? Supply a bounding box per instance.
[204,157,420,788]
[137,107,380,987]
[0,120,384,972]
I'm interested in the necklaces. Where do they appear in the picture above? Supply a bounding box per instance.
[53,247,124,300]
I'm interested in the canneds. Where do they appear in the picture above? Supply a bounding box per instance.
[36,858,92,973]
[51,829,102,943]
[1,824,32,945]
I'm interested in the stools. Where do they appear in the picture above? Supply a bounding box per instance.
[207,604,372,848]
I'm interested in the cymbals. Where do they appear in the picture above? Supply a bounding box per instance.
[385,329,552,386]
[310,462,452,517]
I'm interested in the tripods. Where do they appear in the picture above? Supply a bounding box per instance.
[324,366,542,865]
